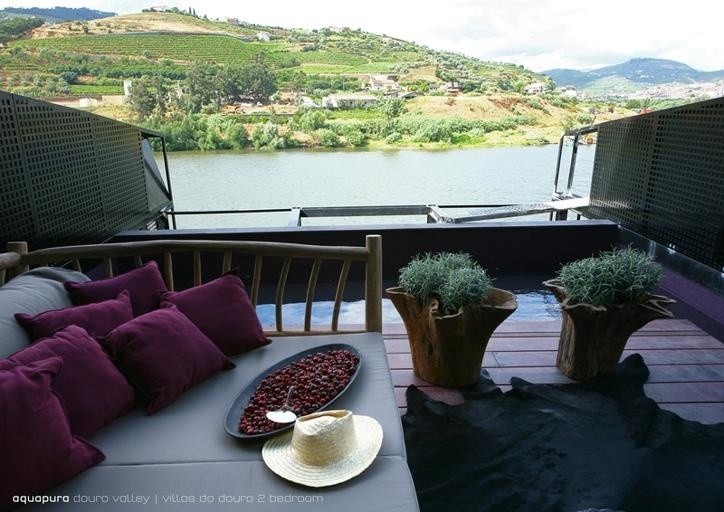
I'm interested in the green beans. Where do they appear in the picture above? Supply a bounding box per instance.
[239,350,359,434]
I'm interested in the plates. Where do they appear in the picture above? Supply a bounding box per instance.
[221,342,362,442]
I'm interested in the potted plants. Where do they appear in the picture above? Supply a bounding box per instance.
[384,248,518,390]
[540,242,677,383]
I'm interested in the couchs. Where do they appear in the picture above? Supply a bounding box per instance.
[0,234,422,512]
